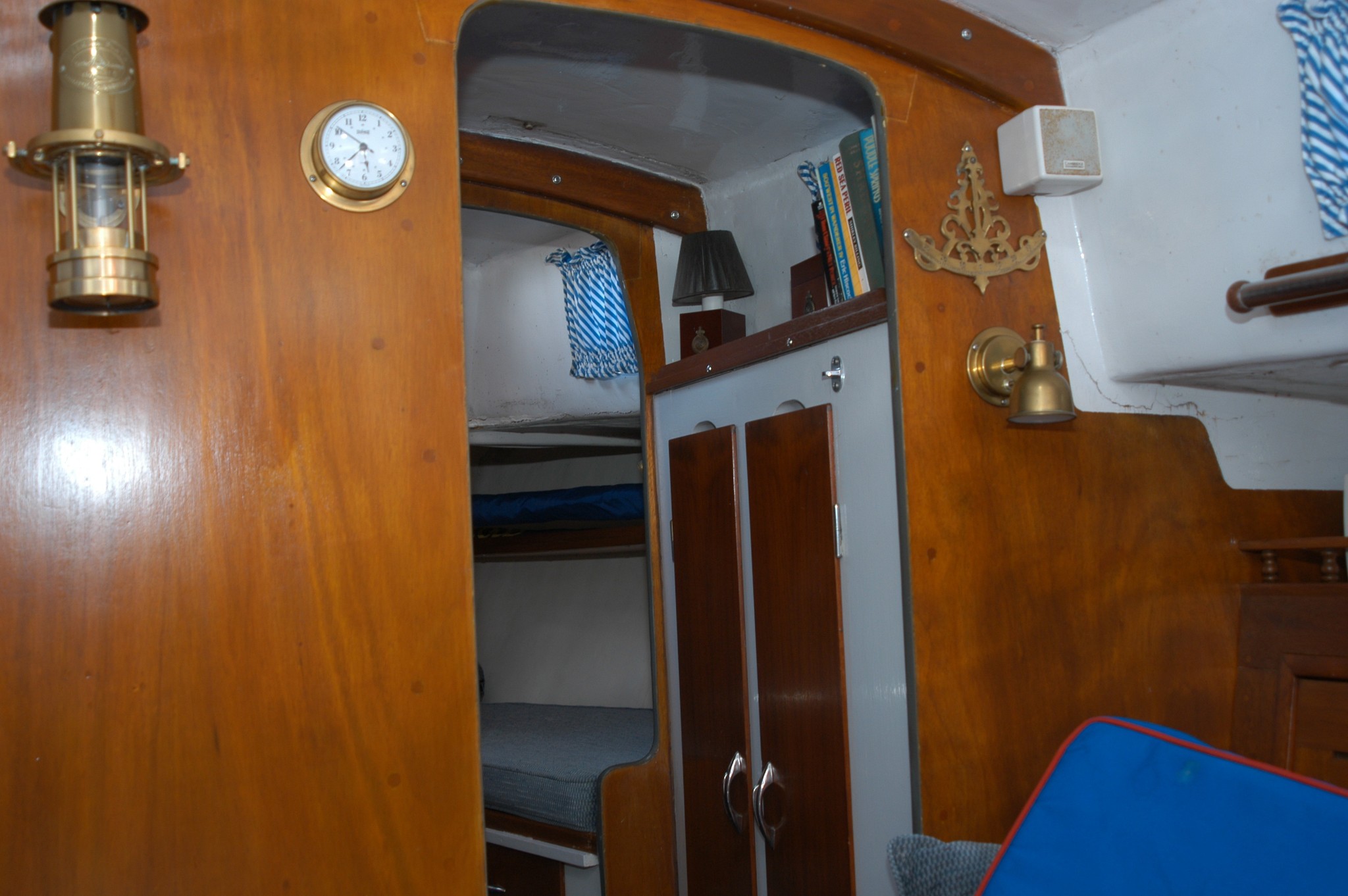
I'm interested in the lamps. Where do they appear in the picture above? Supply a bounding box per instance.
[6,0,187,317]
[671,229,755,360]
[966,324,1077,424]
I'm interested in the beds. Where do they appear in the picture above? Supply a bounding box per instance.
[475,663,656,896]
[467,406,647,527]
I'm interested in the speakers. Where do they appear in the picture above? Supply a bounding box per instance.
[996,105,1102,196]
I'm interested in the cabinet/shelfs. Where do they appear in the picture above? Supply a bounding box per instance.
[623,289,915,896]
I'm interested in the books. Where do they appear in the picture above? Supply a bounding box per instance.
[810,127,884,306]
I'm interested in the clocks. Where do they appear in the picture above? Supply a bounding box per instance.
[300,100,415,214]
